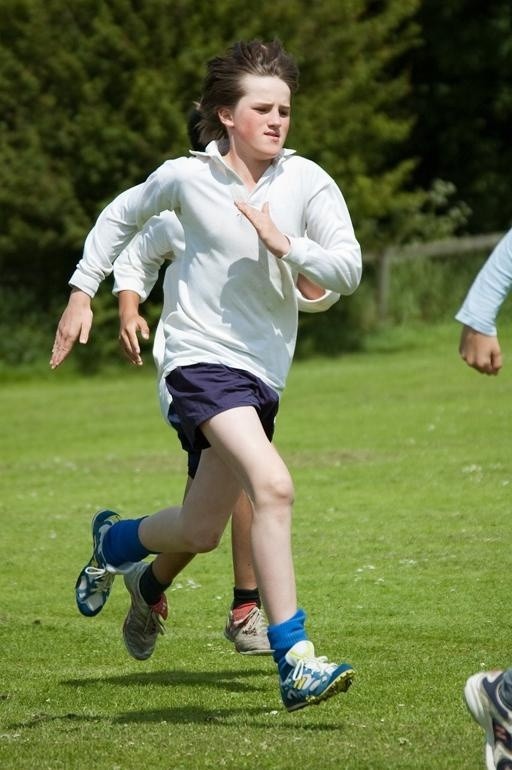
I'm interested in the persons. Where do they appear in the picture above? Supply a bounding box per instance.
[51,37,362,713]
[112,101,342,661]
[453,227,512,770]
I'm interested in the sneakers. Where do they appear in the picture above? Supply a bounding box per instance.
[464,666,512,770]
[75,508,123,618]
[223,604,274,657]
[123,561,168,661]
[278,639,357,713]
